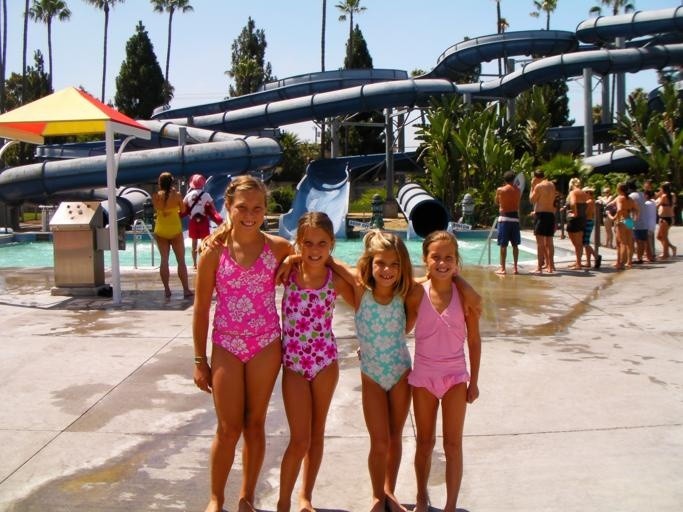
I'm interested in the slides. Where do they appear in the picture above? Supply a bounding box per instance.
[0,7,682,242]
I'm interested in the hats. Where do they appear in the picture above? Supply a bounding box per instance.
[189,174,205,189]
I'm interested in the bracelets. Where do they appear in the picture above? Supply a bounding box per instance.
[194,356,207,365]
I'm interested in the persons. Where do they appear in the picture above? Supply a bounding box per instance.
[181,173,223,271]
[494,170,521,274]
[151,172,194,298]
[357,229,481,512]
[273,228,483,512]
[202,211,355,512]
[526,169,677,276]
[192,174,297,512]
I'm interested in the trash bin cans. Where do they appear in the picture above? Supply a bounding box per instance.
[49,201,111,296]
